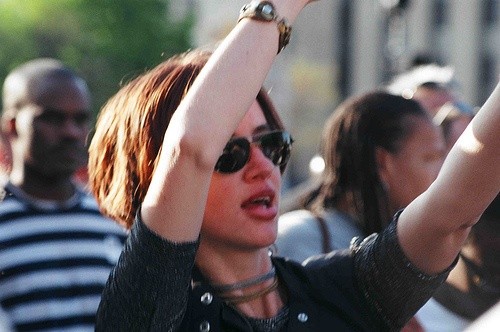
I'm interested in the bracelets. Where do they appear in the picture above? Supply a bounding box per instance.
[237,0,292,55]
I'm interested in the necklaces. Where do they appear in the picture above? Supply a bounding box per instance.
[212,267,279,303]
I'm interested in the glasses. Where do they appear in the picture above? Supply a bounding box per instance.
[213,131,292,174]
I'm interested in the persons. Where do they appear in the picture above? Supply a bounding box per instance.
[269,89,446,332]
[0,57,129,332]
[408,79,500,268]
[87,0,500,332]
